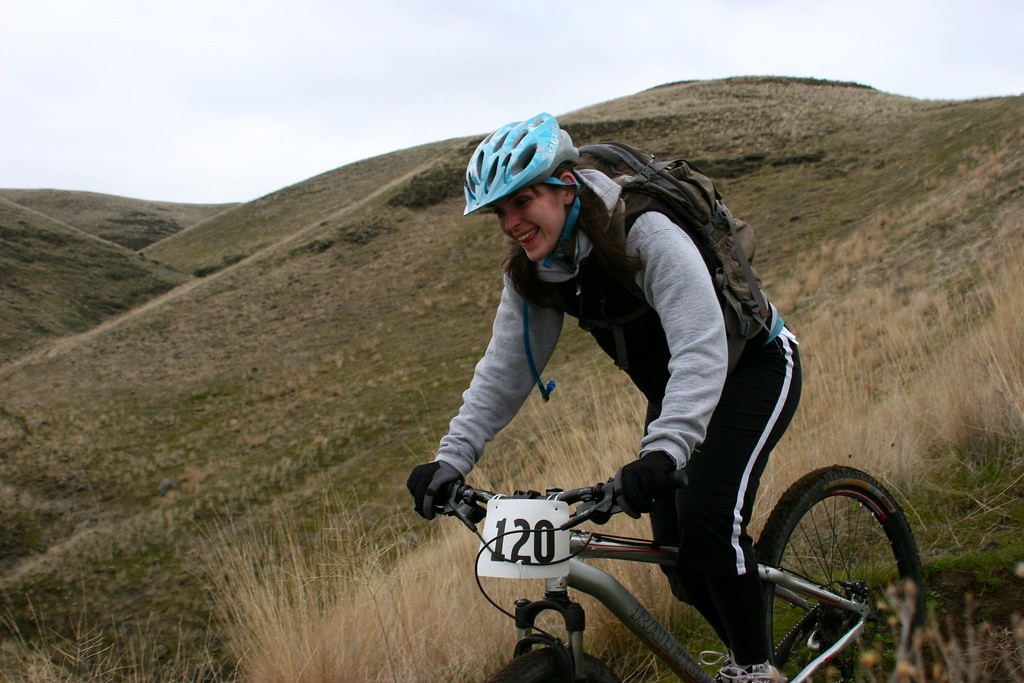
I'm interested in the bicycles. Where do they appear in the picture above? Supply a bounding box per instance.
[434,463,929,682]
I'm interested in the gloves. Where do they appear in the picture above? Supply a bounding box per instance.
[616,450,676,518]
[405,461,463,520]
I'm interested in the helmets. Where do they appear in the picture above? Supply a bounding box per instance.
[461,113,580,218]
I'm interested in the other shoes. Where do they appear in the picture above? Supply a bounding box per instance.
[699,651,789,683]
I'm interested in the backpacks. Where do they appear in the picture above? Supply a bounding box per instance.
[570,140,770,376]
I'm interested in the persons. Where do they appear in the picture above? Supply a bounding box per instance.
[406,148,802,683]
